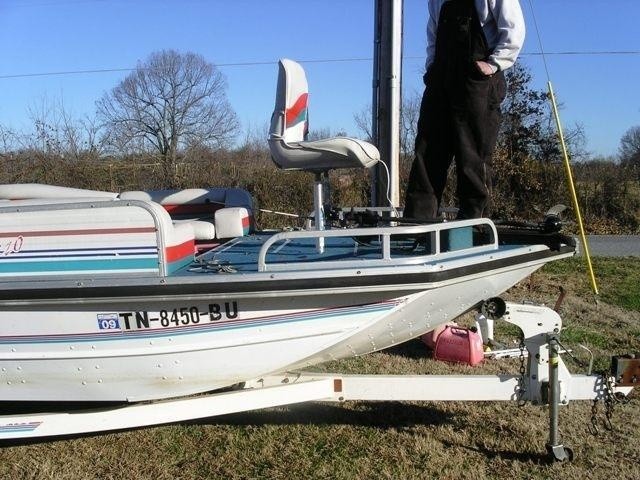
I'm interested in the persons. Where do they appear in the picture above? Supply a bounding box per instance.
[402,0,526,238]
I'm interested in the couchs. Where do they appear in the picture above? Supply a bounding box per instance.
[0,200,195,278]
[120,188,254,240]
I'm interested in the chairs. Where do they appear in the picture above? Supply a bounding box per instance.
[267,57,381,255]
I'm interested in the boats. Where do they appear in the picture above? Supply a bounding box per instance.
[0,56,580,405]
